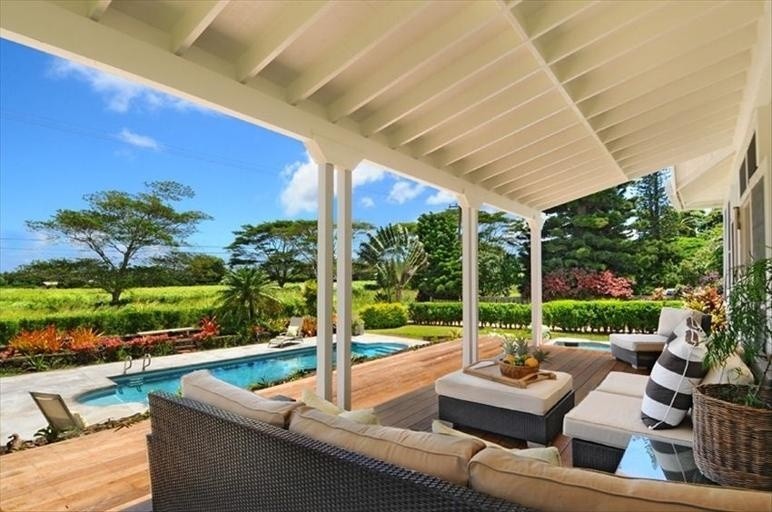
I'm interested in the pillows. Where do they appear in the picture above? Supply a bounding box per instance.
[615,434,665,480]
[432,420,559,465]
[303,394,378,427]
[640,318,711,431]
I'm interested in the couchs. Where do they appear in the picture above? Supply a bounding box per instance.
[571,352,753,487]
[132,387,769,512]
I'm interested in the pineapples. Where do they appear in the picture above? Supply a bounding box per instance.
[500,333,552,367]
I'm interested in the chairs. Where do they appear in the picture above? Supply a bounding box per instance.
[607,306,713,371]
[268,316,307,347]
[28,387,97,436]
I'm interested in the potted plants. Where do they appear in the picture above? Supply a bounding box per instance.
[679,255,771,494]
